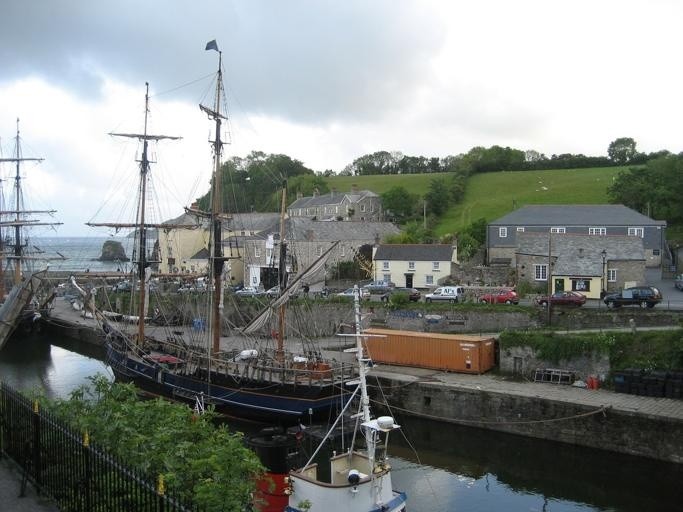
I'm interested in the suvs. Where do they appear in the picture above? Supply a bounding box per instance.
[603,285,663,309]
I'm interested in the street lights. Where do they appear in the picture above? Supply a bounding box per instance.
[602,250,607,298]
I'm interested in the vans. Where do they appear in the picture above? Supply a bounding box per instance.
[426,287,465,303]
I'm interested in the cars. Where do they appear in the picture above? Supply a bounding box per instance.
[537,291,585,307]
[118,276,279,297]
[381,287,421,302]
[337,288,370,301]
[478,288,518,304]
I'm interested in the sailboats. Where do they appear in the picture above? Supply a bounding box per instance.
[70,39,360,426]
[0,116,69,343]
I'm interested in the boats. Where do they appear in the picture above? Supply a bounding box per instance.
[249,286,409,510]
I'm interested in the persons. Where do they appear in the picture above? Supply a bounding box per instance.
[302,284,309,297]
